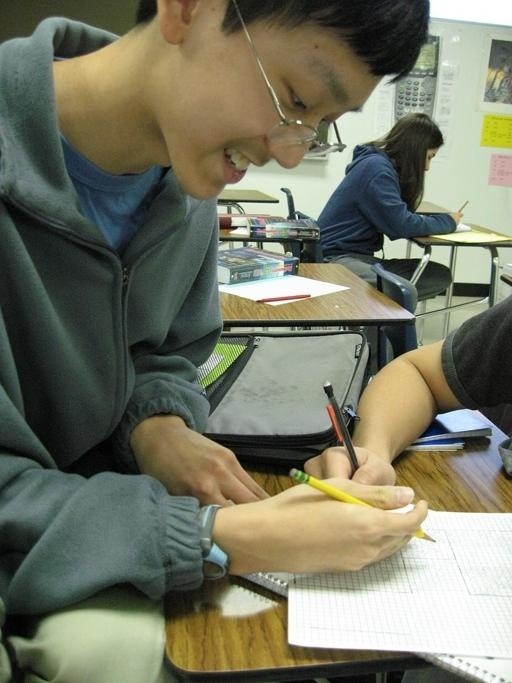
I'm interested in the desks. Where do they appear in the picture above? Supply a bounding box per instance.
[212,189,282,214]
[165,406,511,682]
[213,262,417,399]
[395,200,512,348]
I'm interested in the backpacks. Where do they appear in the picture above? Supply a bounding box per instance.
[190,331,372,476]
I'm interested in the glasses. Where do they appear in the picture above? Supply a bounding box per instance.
[232,1,347,158]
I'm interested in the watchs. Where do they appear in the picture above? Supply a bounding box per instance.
[196,504,230,582]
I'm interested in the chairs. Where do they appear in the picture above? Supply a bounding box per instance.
[283,189,304,219]
[294,209,327,263]
[371,263,420,360]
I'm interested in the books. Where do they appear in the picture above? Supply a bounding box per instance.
[240,571,512,682]
[401,408,493,452]
[216,245,300,286]
[229,213,321,241]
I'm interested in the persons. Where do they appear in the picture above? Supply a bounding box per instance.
[297,112,464,373]
[0,1,431,683]
[301,294,512,488]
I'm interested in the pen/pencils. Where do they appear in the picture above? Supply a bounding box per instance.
[289,467,438,545]
[255,294,311,303]
[458,201,468,213]
[324,383,364,468]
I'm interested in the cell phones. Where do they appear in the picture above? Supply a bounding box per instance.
[394,31,441,127]
